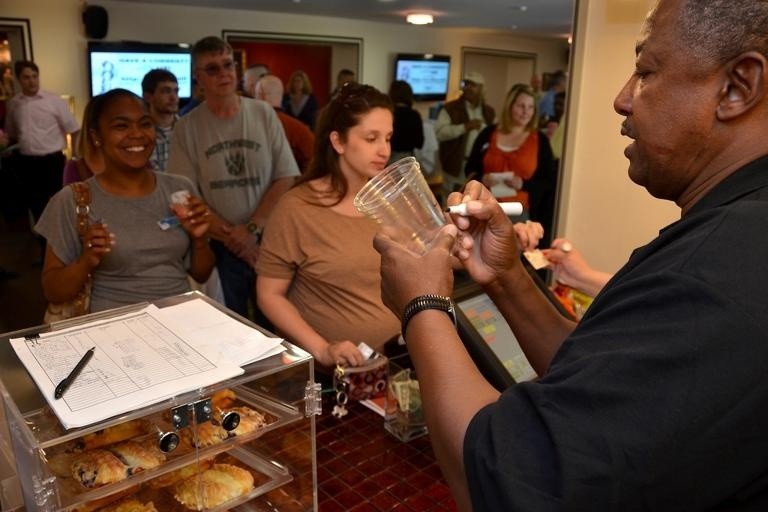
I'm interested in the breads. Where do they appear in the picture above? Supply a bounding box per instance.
[40,384,265,512]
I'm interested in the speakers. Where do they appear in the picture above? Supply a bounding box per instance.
[85,5,108,39]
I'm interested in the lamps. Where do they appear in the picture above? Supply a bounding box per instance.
[406,13,433,26]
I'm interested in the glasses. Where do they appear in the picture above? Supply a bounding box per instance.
[195,62,238,75]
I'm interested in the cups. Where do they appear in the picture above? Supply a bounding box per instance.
[172,190,196,219]
[354,155,462,261]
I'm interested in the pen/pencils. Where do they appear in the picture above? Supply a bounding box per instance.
[444,202,524,217]
[561,241,573,252]
[55,347,96,400]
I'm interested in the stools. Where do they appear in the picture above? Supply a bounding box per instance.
[426,174,446,203]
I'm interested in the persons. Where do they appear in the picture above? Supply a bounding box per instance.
[372,0,768,512]
[540,238,615,299]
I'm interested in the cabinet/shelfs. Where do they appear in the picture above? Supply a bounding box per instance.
[0,290,323,512]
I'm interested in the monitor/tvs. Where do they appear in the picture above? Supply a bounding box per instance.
[449,264,578,390]
[88,41,194,109]
[395,53,450,102]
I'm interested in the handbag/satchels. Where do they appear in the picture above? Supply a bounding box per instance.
[42,180,94,326]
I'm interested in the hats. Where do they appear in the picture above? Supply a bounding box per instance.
[462,72,483,85]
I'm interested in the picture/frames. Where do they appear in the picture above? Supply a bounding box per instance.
[0,17,34,103]
